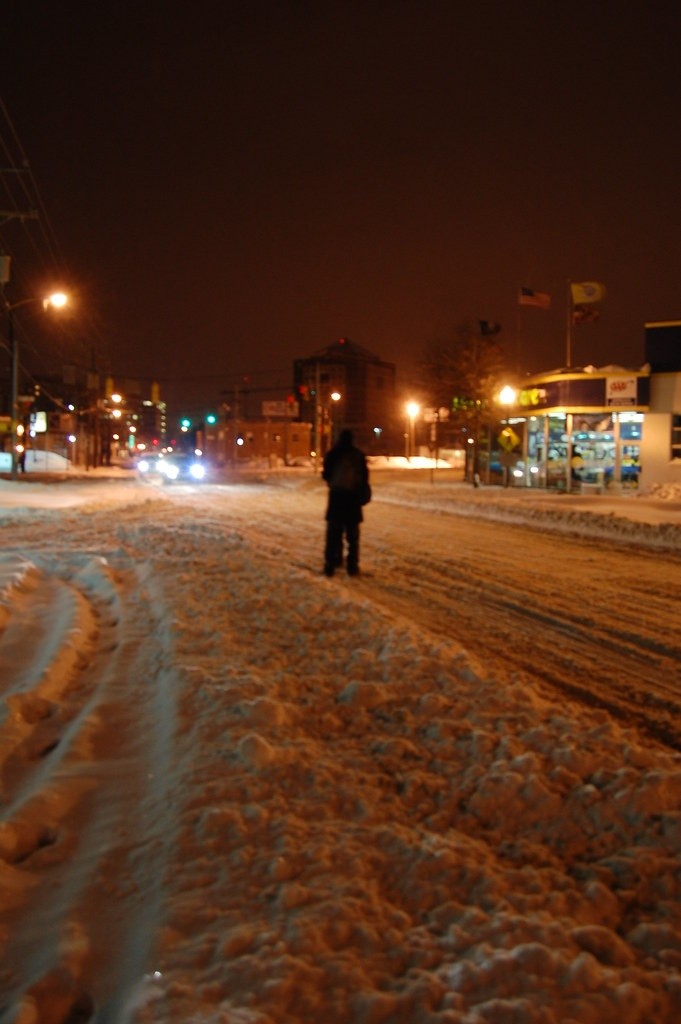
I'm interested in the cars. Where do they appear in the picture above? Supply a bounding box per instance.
[138,452,163,475]
[160,453,204,485]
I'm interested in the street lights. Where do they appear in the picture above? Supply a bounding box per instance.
[6,292,68,475]
[500,384,517,488]
[320,391,341,463]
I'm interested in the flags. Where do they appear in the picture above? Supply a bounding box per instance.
[571,282,606,304]
[572,306,601,327]
[479,320,502,335]
[516,288,551,309]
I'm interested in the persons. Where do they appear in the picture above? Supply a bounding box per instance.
[18,450,25,473]
[321,430,373,577]
